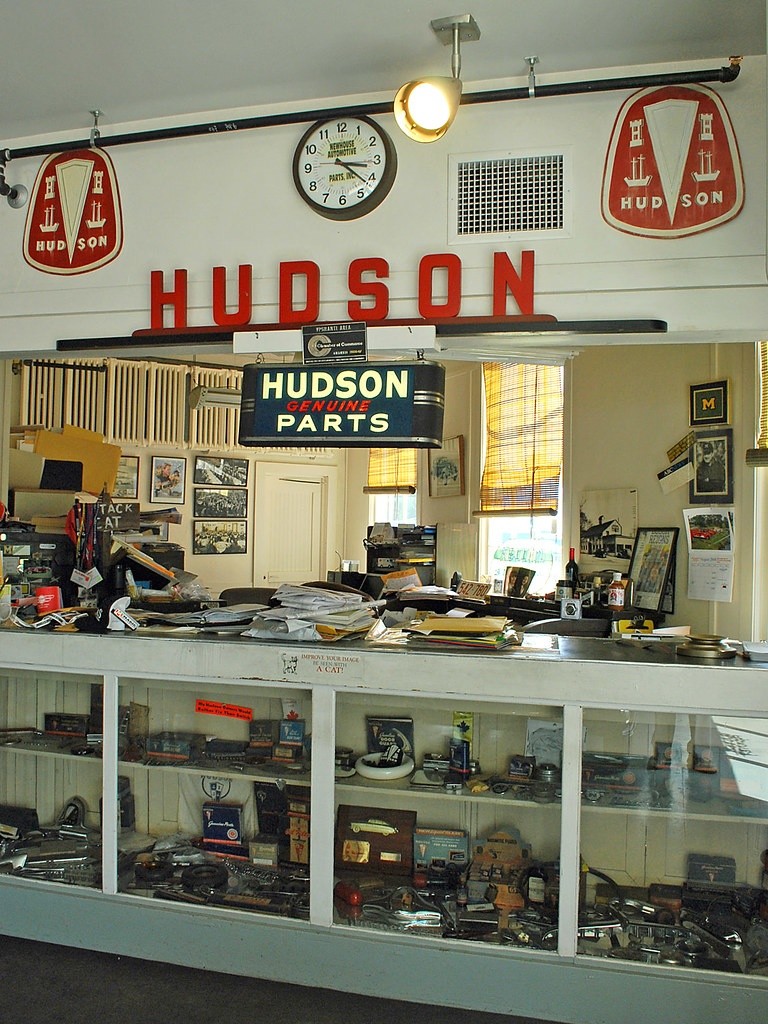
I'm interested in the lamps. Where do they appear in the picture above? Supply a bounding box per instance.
[394,14,481,142]
[190,385,241,409]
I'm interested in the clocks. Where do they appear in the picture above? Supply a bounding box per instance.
[292,114,398,221]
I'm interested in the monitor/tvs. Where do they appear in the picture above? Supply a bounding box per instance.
[359,572,388,600]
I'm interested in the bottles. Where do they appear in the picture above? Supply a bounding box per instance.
[607,572,625,611]
[565,548,579,599]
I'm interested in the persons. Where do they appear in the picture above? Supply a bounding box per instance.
[196,462,246,485]
[195,490,245,515]
[508,571,529,597]
[696,443,725,492]
[155,463,172,484]
[195,526,245,552]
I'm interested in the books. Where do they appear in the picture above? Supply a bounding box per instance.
[402,614,517,650]
[240,583,376,642]
[153,603,270,627]
[400,525,436,546]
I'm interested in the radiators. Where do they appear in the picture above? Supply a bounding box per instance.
[10,356,333,459]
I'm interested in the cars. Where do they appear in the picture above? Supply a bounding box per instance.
[349,818,399,837]
[690,529,716,539]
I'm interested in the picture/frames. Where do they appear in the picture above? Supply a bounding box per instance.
[194,456,249,486]
[193,520,248,554]
[150,456,187,505]
[193,488,248,518]
[687,429,735,504]
[427,435,466,498]
[110,455,140,499]
[688,378,733,427]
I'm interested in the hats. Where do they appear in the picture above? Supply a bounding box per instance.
[702,443,713,453]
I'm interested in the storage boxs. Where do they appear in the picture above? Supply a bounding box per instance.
[248,832,290,865]
[526,873,597,908]
[413,824,470,877]
[45,712,90,738]
[243,717,305,760]
[687,853,736,895]
[201,801,244,845]
[146,730,207,760]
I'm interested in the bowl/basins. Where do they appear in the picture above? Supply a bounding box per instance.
[354,752,415,780]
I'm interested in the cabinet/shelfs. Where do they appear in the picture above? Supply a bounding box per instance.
[1,629,768,1024]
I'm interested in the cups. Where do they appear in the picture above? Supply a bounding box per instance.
[554,580,574,603]
[0,599,12,625]
